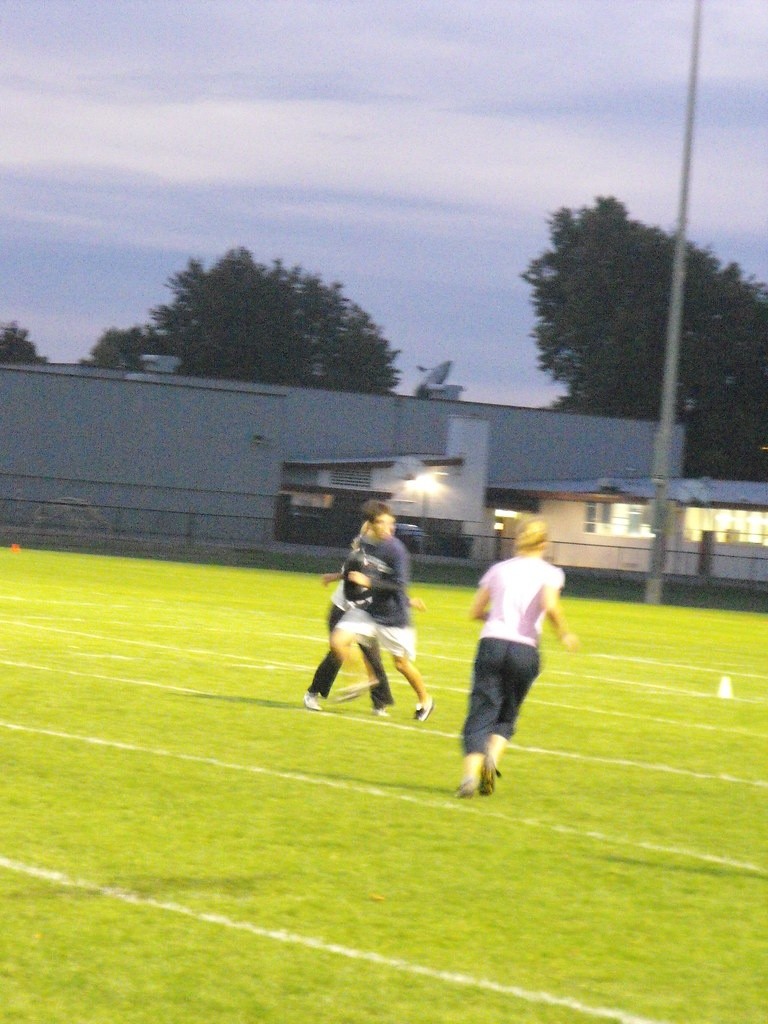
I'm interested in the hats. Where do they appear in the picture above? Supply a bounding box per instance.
[513,517,548,557]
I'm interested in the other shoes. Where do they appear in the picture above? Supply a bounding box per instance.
[454,781,475,800]
[304,692,323,710]
[413,696,434,721]
[335,680,381,703]
[476,761,502,796]
[371,704,391,717]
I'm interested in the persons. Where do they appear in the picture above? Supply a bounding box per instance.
[304,501,435,720]
[454,516,576,800]
